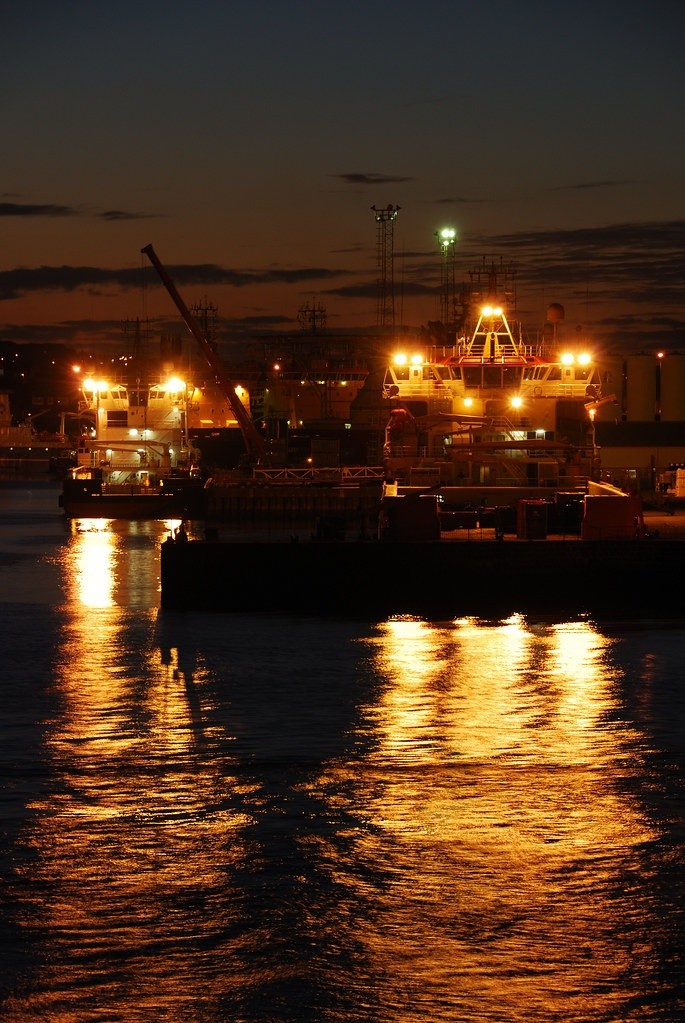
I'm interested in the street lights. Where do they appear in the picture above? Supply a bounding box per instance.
[85,380,108,440]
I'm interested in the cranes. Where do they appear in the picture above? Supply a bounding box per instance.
[137,242,291,474]
